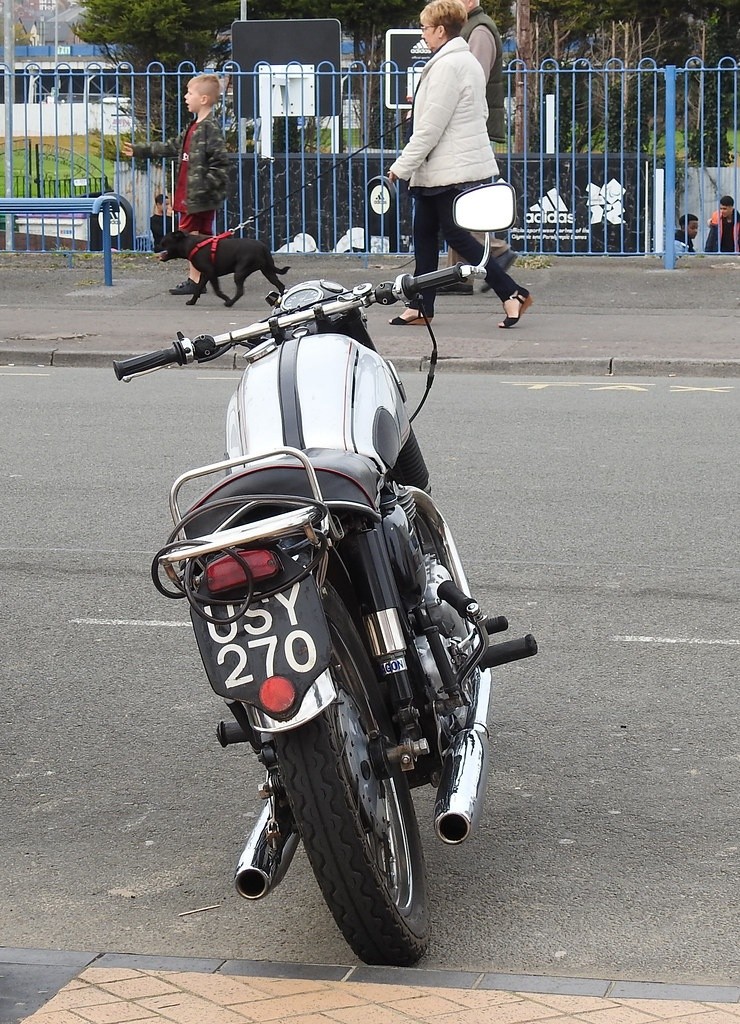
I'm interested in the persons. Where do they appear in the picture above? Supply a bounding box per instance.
[436,0,518,296]
[704,196,740,256]
[675,214,698,255]
[387,0,533,328]
[121,75,231,295]
[150,194,179,245]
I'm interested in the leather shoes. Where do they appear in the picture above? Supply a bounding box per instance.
[436,282,474,295]
[480,249,519,293]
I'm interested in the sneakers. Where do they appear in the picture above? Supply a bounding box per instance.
[169,278,207,295]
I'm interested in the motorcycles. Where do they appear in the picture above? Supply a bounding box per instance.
[113,183,539,967]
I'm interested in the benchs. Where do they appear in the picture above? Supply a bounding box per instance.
[0,194,120,287]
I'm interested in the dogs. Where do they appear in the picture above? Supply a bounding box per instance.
[154,230,290,306]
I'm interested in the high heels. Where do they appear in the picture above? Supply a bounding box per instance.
[498,284,533,328]
[389,305,434,325]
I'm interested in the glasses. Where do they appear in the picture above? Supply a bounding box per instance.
[420,24,432,32]
